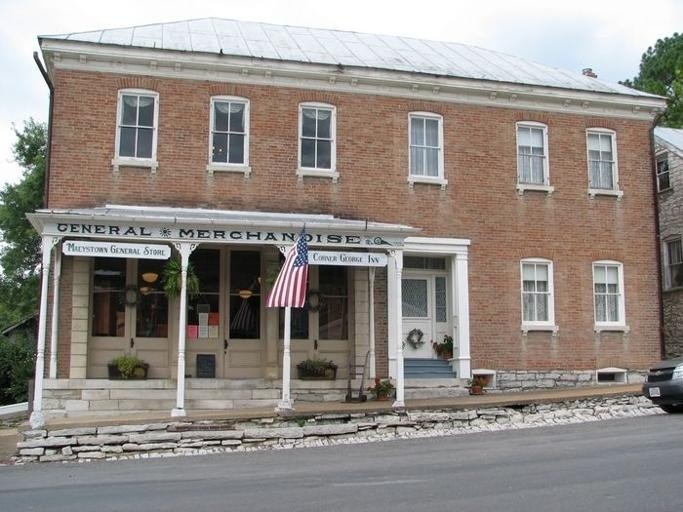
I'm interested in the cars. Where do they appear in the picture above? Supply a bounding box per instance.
[642,356,683,413]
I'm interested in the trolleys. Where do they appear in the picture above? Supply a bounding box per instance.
[345,348,371,403]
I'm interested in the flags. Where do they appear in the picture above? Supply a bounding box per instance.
[264,229,310,312]
[229,281,260,333]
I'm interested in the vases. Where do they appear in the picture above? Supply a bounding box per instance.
[443,353,453,359]
[377,394,388,401]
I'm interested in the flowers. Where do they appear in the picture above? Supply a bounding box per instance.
[433,335,453,356]
[370,377,393,397]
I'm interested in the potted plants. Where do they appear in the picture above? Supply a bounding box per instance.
[108,356,149,380]
[467,380,488,394]
[296,357,338,380]
